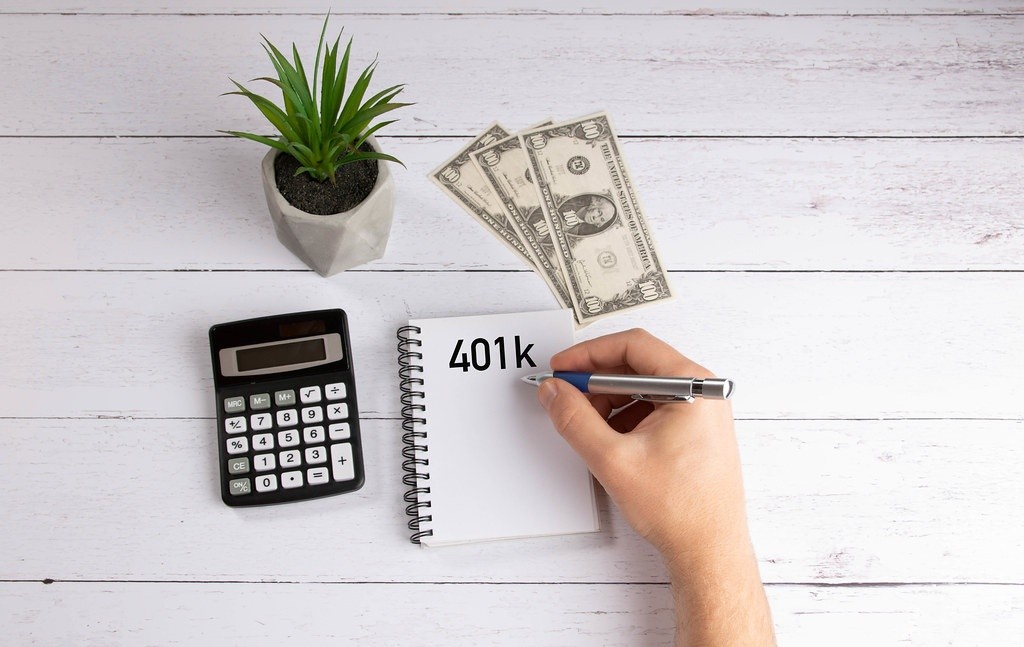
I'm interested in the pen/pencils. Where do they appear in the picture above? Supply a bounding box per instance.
[520,371,734,404]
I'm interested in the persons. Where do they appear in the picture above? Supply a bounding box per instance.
[537,328,778,647]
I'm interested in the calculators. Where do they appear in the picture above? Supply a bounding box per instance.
[208,308,366,508]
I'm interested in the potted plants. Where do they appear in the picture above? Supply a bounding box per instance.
[213,8,417,277]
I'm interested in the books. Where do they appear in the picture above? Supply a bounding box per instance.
[396,310,600,548]
